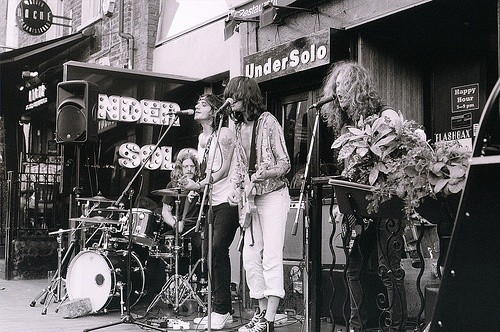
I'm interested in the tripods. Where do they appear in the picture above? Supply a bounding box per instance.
[25,108,207,332]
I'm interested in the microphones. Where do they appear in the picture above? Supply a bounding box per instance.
[308,93,337,108]
[216,97,234,113]
[167,108,195,115]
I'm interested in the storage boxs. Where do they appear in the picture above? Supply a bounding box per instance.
[249,260,305,316]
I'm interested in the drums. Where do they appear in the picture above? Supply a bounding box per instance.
[148,236,192,259]
[65,246,145,313]
[121,206,163,248]
[108,238,134,251]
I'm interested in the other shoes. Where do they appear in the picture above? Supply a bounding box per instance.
[193,315,233,324]
[204,311,230,329]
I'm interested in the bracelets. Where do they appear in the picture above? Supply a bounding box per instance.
[198,182,201,189]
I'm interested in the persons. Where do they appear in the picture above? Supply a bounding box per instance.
[320,61,408,332]
[178,94,239,329]
[161,148,208,316]
[223,75,291,332]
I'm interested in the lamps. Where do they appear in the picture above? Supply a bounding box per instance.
[224,16,286,41]
[260,1,315,30]
[24,64,63,90]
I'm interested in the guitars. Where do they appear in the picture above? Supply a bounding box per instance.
[237,158,271,229]
[177,174,209,218]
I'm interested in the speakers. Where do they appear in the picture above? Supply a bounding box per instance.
[56,79,89,142]
[282,203,349,265]
[275,265,352,325]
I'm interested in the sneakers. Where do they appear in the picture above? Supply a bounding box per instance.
[238,307,274,332]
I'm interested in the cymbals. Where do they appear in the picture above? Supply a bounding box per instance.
[74,193,115,204]
[92,208,128,212]
[151,186,191,197]
[68,214,122,226]
